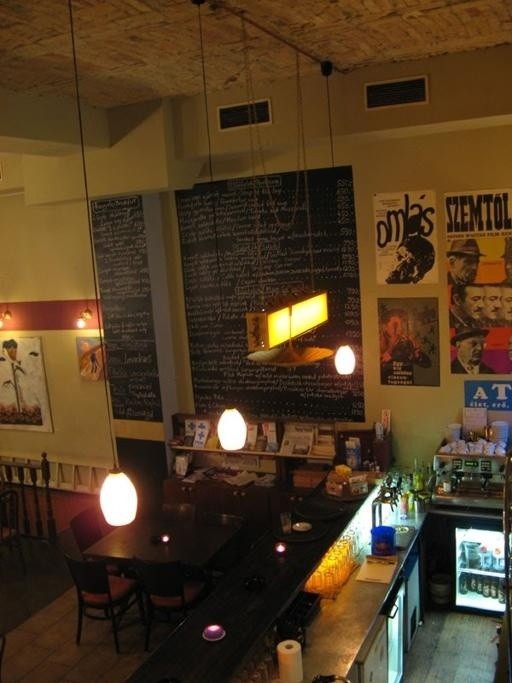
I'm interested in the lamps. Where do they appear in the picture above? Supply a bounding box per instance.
[317,57,360,377]
[238,15,333,352]
[63,3,138,527]
[194,0,250,453]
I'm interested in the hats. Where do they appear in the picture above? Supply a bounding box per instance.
[447,239,487,256]
[501,237,512,256]
[450,325,489,346]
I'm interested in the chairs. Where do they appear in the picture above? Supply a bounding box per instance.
[135,558,210,654]
[64,555,142,657]
[0,489,25,555]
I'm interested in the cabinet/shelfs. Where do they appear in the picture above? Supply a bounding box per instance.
[168,412,339,462]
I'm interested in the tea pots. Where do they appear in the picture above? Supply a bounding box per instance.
[441,423,462,442]
[496,447,505,456]
[496,441,506,448]
[469,447,482,455]
[483,447,494,454]
[469,442,483,450]
[485,442,496,449]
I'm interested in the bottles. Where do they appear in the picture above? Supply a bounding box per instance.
[413,457,424,492]
[483,577,490,597]
[464,573,469,590]
[498,580,505,603]
[471,573,476,592]
[424,463,435,492]
[477,575,483,594]
[460,573,467,594]
[490,578,498,598]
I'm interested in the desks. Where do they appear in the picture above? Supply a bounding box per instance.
[79,504,248,578]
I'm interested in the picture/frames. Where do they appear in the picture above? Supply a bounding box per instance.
[0,336,56,434]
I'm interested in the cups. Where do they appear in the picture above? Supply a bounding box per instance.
[443,480,451,492]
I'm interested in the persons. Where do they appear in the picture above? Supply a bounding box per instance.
[380,303,432,385]
[451,285,486,329]
[481,286,503,330]
[450,322,496,374]
[500,285,511,326]
[500,237,511,283]
[447,237,486,285]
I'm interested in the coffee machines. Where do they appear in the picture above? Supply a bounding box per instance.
[431,455,506,509]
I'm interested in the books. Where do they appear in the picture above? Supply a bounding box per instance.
[356,554,399,586]
[390,523,416,552]
[170,414,337,491]
